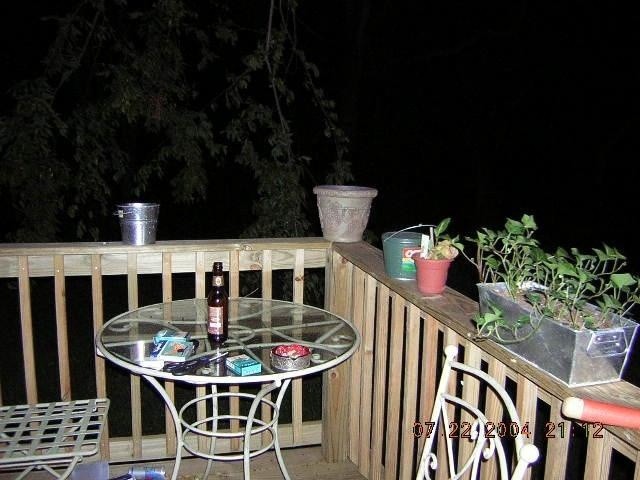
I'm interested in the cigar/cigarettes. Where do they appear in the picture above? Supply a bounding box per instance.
[281,350,297,357]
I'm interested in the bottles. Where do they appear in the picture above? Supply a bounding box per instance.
[206,261,229,342]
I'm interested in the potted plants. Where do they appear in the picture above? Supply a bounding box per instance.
[441,211,640,388]
[411,216,465,294]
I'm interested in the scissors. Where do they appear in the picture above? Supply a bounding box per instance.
[163,352,228,376]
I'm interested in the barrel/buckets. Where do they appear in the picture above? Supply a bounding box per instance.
[382,222,438,280]
[113,202,161,246]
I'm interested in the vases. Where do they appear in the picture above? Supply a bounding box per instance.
[312,185,378,242]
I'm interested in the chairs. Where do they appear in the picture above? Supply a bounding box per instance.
[417,344,542,480]
[0,397,111,480]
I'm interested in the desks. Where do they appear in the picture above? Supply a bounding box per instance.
[95,297,362,480]
